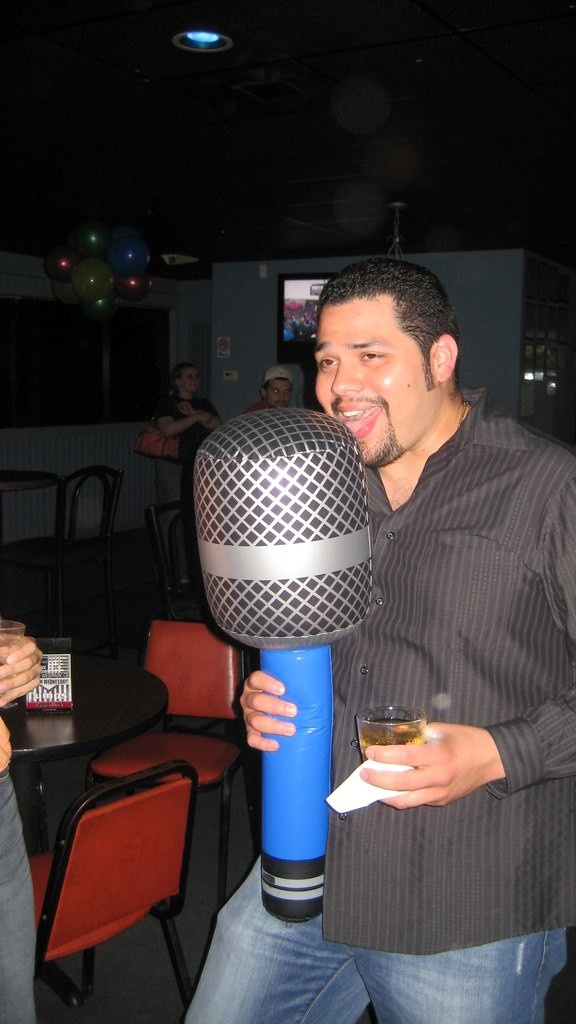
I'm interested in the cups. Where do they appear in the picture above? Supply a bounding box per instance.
[0,620,25,708]
[356,706,427,762]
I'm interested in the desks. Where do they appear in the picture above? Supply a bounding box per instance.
[0,654,170,1009]
[0,468,60,492]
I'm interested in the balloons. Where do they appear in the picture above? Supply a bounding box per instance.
[43,220,152,322]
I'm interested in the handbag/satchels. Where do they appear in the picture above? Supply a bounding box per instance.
[133,422,180,464]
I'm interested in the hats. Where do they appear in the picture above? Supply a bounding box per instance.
[263,365,294,388]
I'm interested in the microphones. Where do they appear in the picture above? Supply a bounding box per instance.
[195,408,372,922]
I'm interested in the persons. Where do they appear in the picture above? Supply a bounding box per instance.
[0,617,43,1024]
[158,364,292,501]
[283,303,318,341]
[183,259,576,1024]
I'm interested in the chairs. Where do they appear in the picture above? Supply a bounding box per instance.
[146,501,214,625]
[85,620,256,910]
[29,758,199,1009]
[0,466,124,639]
